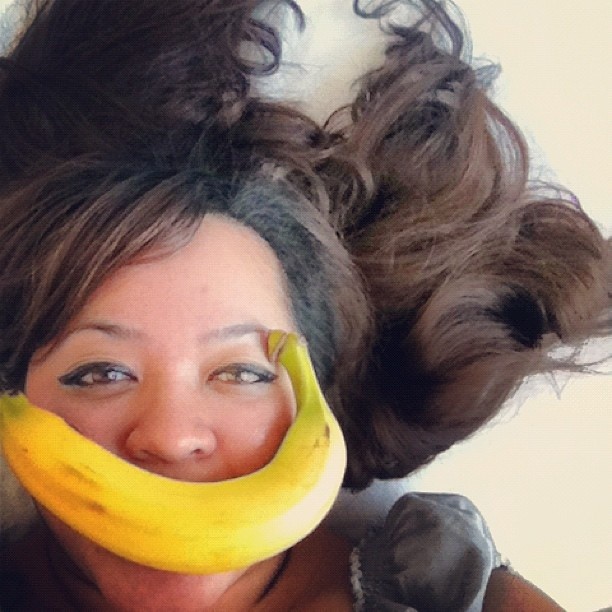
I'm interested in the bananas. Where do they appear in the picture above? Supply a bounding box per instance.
[1,326,350,573]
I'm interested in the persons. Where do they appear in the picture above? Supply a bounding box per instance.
[0,0,612,609]
